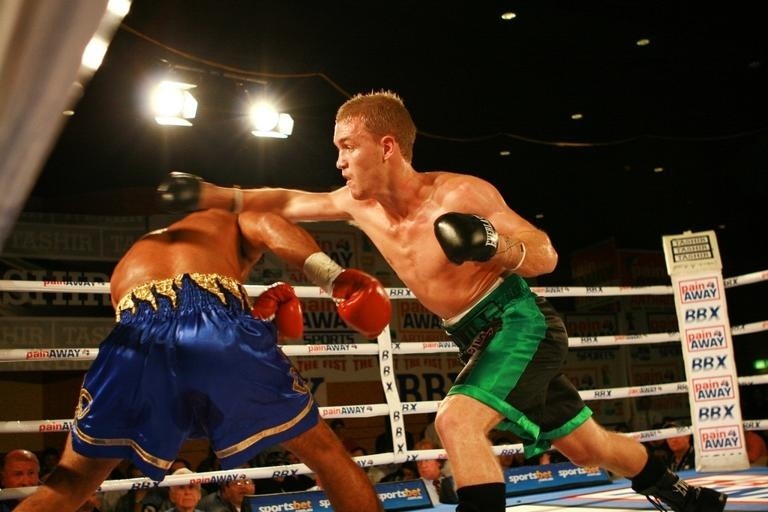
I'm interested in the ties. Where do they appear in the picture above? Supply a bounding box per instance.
[432,478,441,496]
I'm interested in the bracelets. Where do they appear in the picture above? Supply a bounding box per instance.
[506,239,529,273]
[232,186,243,213]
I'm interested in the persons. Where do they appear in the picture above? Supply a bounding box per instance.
[10,209,397,511]
[0,416,419,512]
[157,88,729,512]
[414,411,768,504]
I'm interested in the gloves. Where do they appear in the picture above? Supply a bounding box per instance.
[153,170,203,213]
[332,265,391,338]
[250,281,305,343]
[432,210,501,266]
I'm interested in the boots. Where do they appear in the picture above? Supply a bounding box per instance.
[632,444,729,511]
